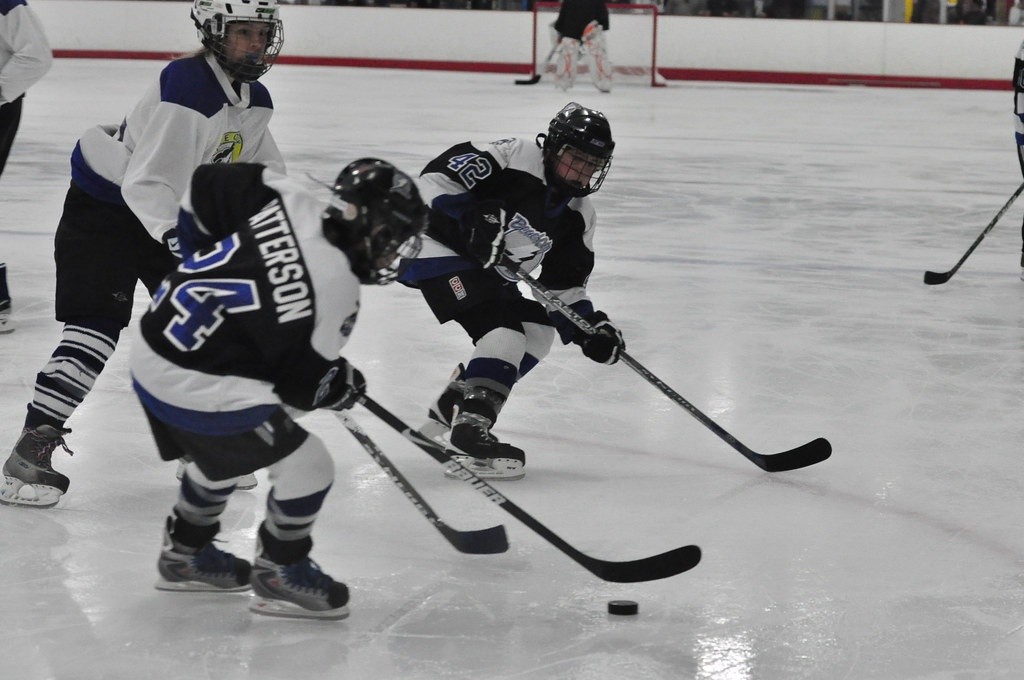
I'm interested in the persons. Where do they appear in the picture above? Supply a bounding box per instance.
[618,0,1024,26]
[1012,39,1024,281]
[553,0,614,92]
[126,158,432,619]
[0,0,287,510]
[394,103,626,479]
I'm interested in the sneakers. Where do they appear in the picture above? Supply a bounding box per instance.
[153,520,252,593]
[447,399,523,476]
[249,523,349,622]
[0,260,16,334]
[420,363,467,452]
[1,420,69,505]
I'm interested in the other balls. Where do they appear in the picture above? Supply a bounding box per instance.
[605,596,643,619]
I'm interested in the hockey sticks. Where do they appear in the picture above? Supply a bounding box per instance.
[333,403,513,560]
[513,39,560,88]
[494,252,837,478]
[352,391,709,587]
[919,184,1024,291]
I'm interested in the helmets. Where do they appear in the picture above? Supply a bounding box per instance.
[190,1,279,79]
[549,102,614,198]
[321,158,431,286]
